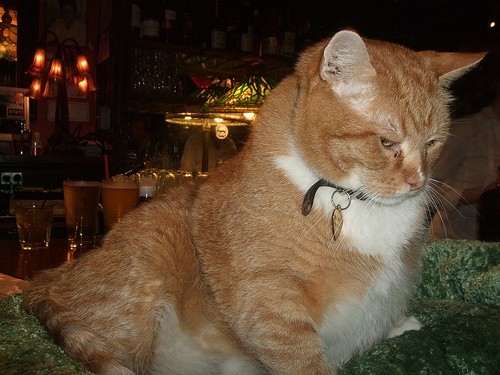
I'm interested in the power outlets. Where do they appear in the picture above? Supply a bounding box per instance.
[1,172,23,185]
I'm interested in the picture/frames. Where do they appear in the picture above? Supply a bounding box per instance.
[45,0,89,48]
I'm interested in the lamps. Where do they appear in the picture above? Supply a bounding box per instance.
[164,66,248,173]
[23,31,97,146]
[133,42,172,104]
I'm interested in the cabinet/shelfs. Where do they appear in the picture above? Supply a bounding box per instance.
[110,0,294,177]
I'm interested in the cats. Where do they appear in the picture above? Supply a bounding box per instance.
[19,29,488,375]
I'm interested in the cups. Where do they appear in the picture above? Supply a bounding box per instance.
[63,181,99,248]
[15,205,54,250]
[102,168,209,229]
